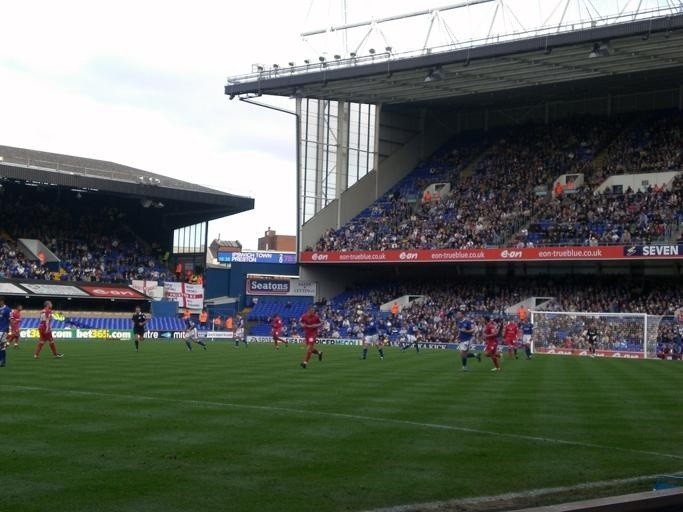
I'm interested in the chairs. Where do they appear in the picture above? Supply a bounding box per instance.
[223,106,683,355]
[2,217,223,355]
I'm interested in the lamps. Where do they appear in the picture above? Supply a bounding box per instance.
[424,67,440,82]
[289,85,305,100]
[589,42,604,58]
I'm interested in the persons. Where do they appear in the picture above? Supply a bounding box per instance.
[303,105,682,253]
[34,300,65,360]
[226,315,233,330]
[199,310,208,326]
[7,304,23,346]
[185,318,208,351]
[0,295,13,368]
[233,274,682,372]
[0,194,203,285]
[183,308,191,319]
[214,315,221,329]
[132,305,147,352]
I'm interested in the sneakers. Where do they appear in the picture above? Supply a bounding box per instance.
[300,363,307,368]
[318,352,322,361]
[53,354,64,358]
[458,353,503,372]
